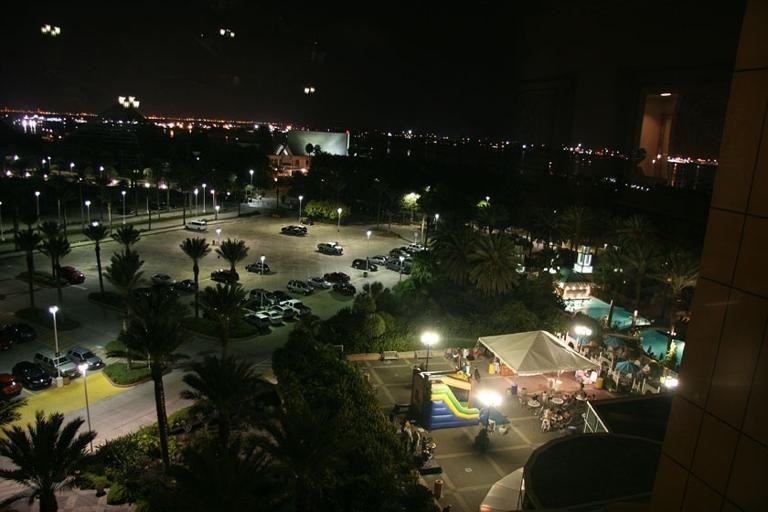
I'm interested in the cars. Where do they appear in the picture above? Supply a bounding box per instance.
[136,270,196,302]
[352,244,430,274]
[242,289,311,328]
[281,225,306,236]
[60,266,86,285]
[1,321,36,353]
[0,346,102,397]
[299,217,313,226]
[286,272,356,296]
[246,263,268,275]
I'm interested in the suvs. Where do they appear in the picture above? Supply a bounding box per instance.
[209,269,241,284]
[317,243,343,255]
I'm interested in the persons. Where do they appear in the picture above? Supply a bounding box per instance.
[388,404,420,455]
[519,383,597,432]
[444,346,503,383]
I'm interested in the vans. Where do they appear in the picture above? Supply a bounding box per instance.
[183,220,206,232]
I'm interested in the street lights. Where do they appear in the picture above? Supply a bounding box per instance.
[49,306,64,387]
[122,191,126,225]
[77,361,94,456]
[484,395,493,434]
[85,201,92,224]
[367,230,372,255]
[298,194,303,216]
[250,169,254,185]
[34,190,41,222]
[202,184,207,212]
[194,189,200,217]
[576,325,590,354]
[336,208,343,231]
[422,328,440,371]
[145,182,150,215]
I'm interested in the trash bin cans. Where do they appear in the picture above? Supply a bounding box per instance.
[363,272,367,278]
